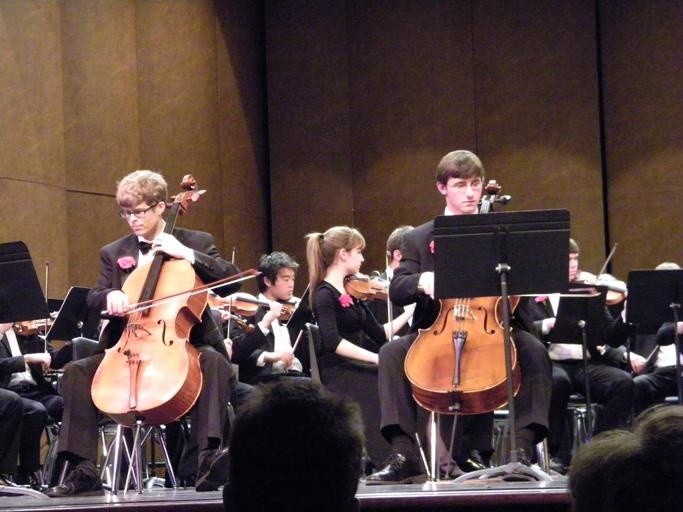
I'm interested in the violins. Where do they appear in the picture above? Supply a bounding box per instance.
[207,290,295,320]
[570,270,629,306]
[12,312,59,336]
[344,272,391,303]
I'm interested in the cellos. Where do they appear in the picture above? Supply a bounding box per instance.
[404,180,520,415]
[90,174,208,427]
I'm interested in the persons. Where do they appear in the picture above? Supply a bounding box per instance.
[47,170,243,497]
[567,403,683,512]
[366,149,553,485]
[223,380,365,512]
[526,239,683,467]
[164,226,495,487]
[0,322,63,486]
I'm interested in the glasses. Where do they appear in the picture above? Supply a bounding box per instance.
[117,201,158,219]
[454,182,482,190]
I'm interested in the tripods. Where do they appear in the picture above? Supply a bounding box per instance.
[453,272,553,483]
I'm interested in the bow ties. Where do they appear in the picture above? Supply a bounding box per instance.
[137,241,153,255]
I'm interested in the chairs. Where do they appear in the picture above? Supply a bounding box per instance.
[55,309,231,491]
[304,322,374,480]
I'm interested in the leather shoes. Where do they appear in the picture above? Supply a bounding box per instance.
[506,447,532,481]
[195,448,230,491]
[47,464,105,498]
[365,454,426,486]
